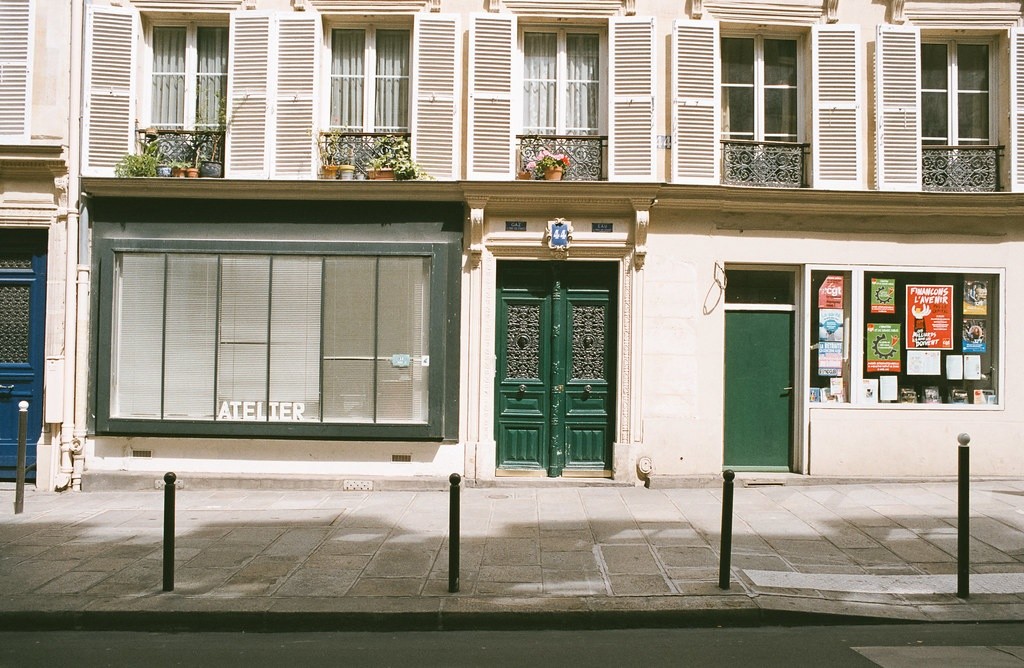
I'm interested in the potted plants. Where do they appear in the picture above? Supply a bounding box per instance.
[114,84,250,178]
[306,128,437,181]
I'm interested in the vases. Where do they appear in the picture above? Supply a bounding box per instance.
[544,166,563,180]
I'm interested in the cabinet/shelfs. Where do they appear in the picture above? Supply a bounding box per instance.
[518,171,531,179]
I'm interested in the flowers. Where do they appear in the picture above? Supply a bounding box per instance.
[523,147,570,175]
